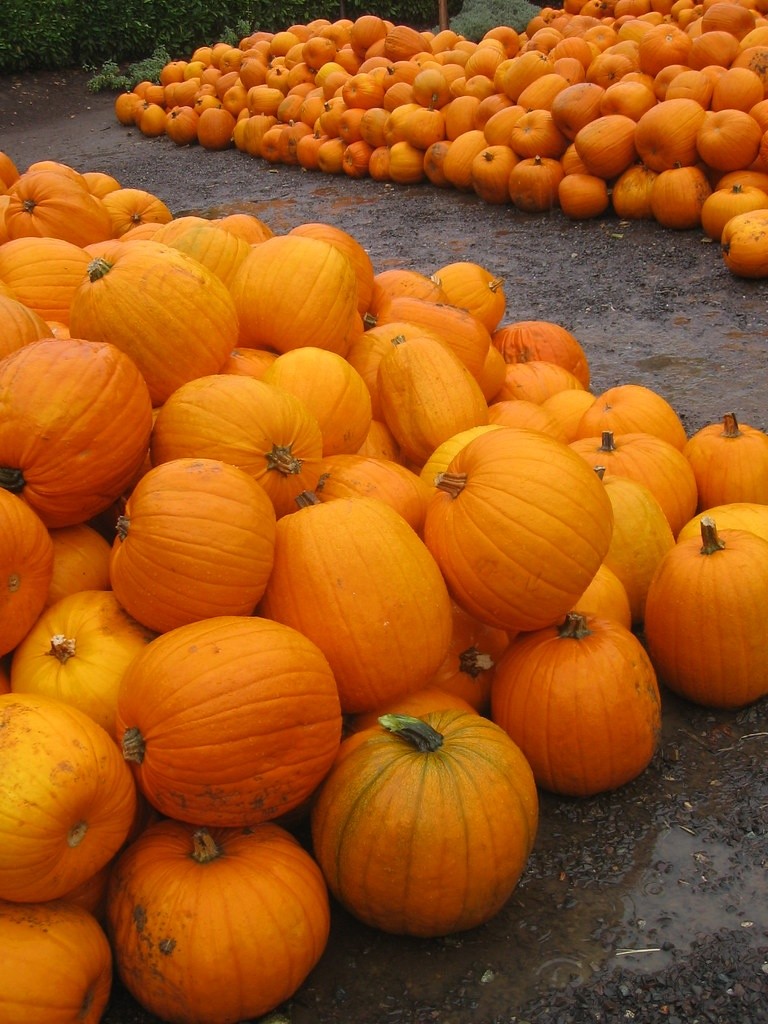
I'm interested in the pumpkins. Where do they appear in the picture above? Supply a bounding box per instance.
[0,152,768,1024]
[116,0,768,280]
